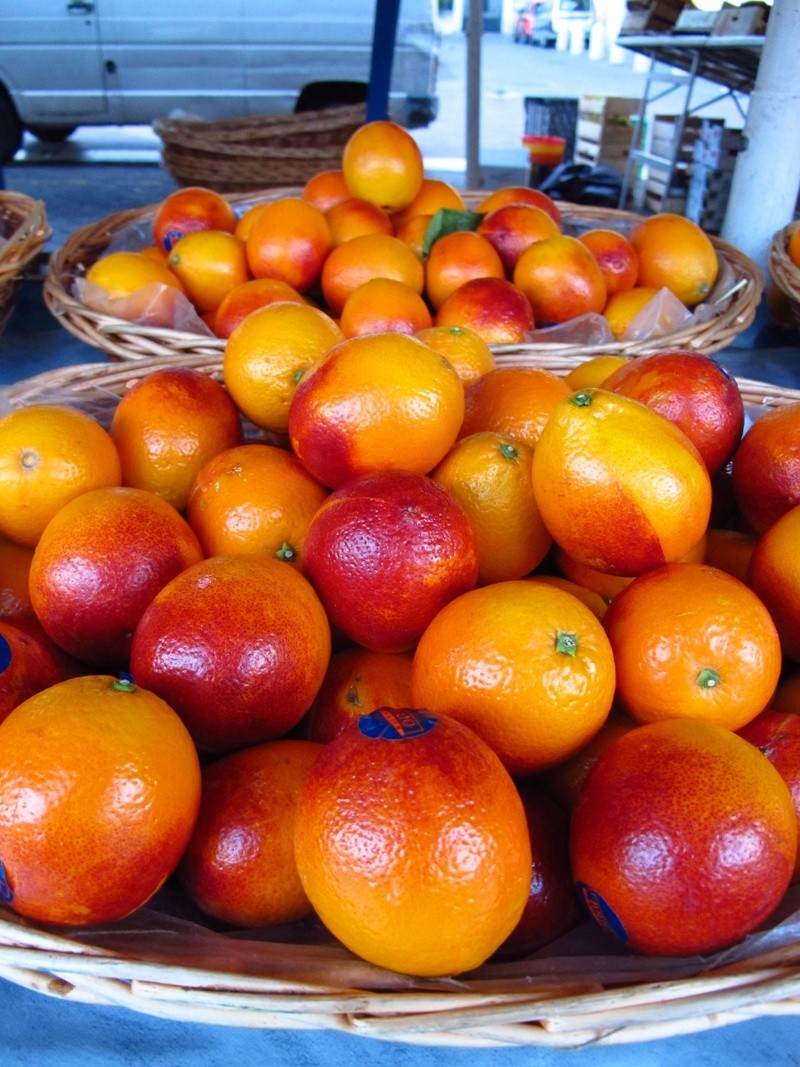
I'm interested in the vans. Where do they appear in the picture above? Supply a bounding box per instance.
[0,0,443,156]
[529,0,602,44]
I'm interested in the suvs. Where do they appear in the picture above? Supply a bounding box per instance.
[512,0,549,43]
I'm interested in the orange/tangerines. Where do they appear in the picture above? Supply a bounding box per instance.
[1,120,800,979]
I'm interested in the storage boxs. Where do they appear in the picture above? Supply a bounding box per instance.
[576,93,642,145]
[649,114,728,164]
[644,164,692,216]
[669,6,719,38]
[572,140,636,188]
[620,0,694,36]
[712,0,769,36]
